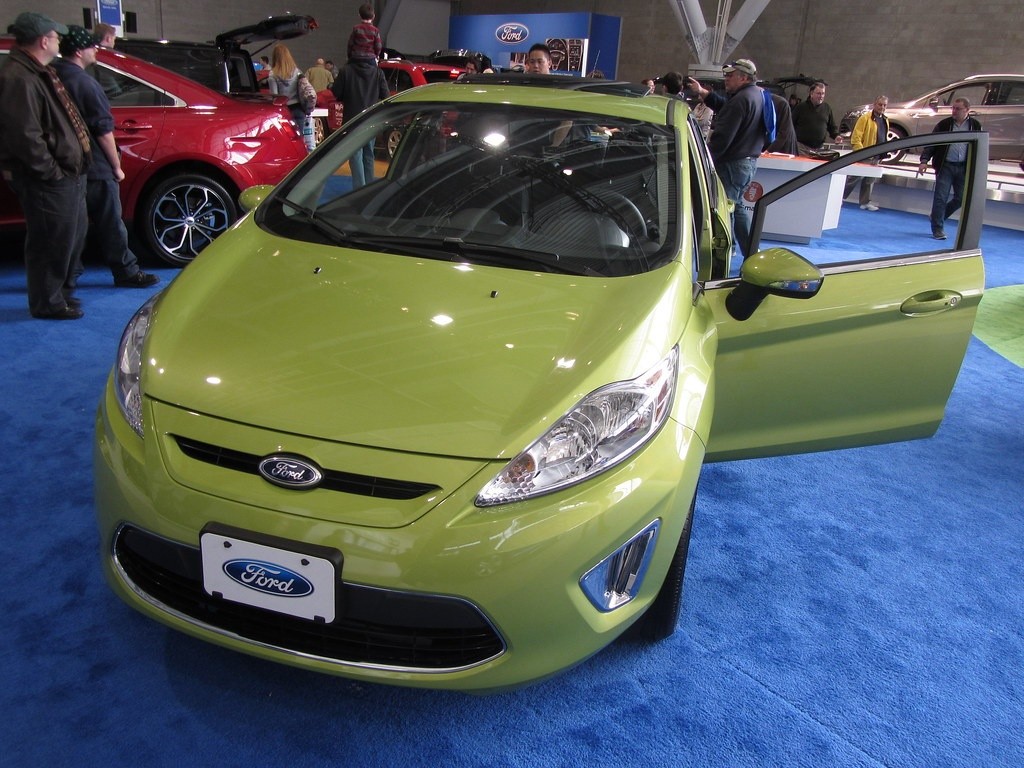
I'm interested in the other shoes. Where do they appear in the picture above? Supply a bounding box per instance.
[64,297,82,308]
[860,200,879,211]
[928,215,932,222]
[114,271,160,288]
[32,305,84,319]
[932,230,948,239]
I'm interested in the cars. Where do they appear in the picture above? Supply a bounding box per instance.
[315,60,474,159]
[0,36,306,266]
[95,71,989,692]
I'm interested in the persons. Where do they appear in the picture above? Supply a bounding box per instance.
[55,25,159,289]
[919,97,983,238]
[0,11,90,320]
[246,4,843,257]
[85,23,127,105]
[840,94,890,211]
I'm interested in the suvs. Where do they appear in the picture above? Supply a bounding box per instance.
[838,73,1024,171]
[114,14,319,99]
[643,66,829,101]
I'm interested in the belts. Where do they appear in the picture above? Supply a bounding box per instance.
[745,156,758,160]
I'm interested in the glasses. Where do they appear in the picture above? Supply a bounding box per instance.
[722,71,733,77]
[949,106,966,111]
[43,35,63,45]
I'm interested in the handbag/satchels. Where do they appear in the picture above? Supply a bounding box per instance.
[302,117,316,151]
[801,146,841,161]
[298,74,317,116]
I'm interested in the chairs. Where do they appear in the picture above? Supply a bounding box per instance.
[599,131,649,195]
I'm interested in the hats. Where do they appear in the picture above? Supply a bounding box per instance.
[58,24,104,53]
[8,12,70,42]
[95,23,116,37]
[723,59,756,75]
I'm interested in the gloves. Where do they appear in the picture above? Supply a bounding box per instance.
[686,77,703,94]
[759,89,777,143]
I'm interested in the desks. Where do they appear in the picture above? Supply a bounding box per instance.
[742,155,885,245]
